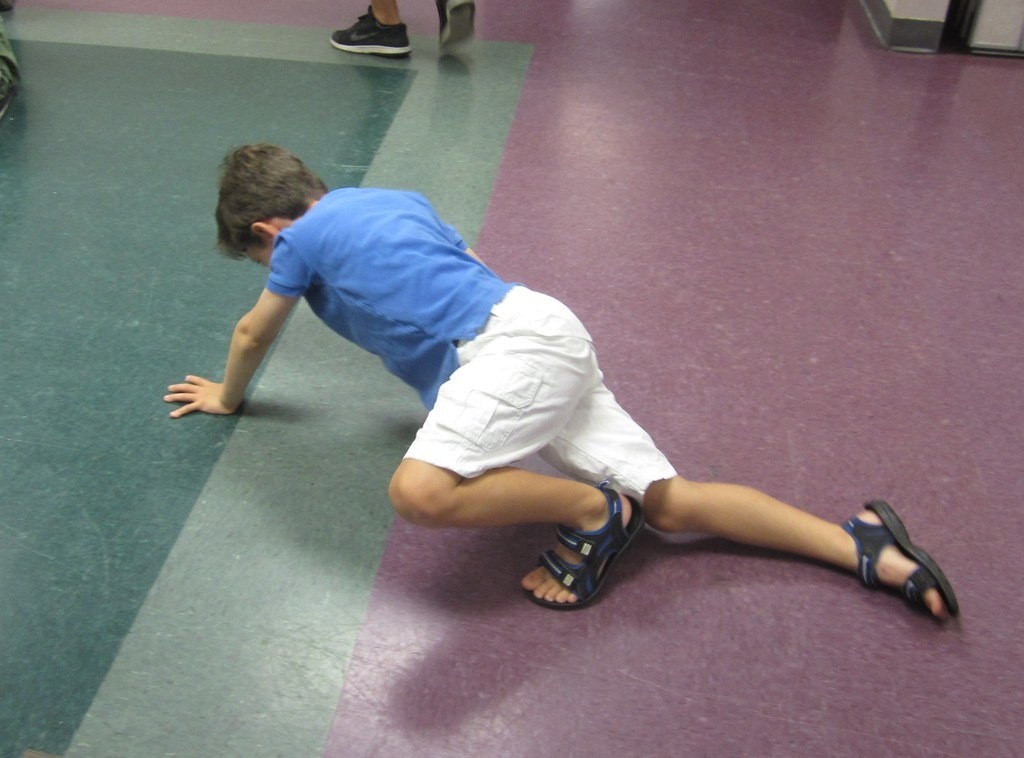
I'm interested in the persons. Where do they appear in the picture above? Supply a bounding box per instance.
[330,0,475,56]
[165,145,960,621]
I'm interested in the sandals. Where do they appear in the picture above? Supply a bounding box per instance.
[843,500,959,618]
[525,480,644,610]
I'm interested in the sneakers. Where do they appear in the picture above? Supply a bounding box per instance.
[436,0,475,51]
[329,3,411,55]
